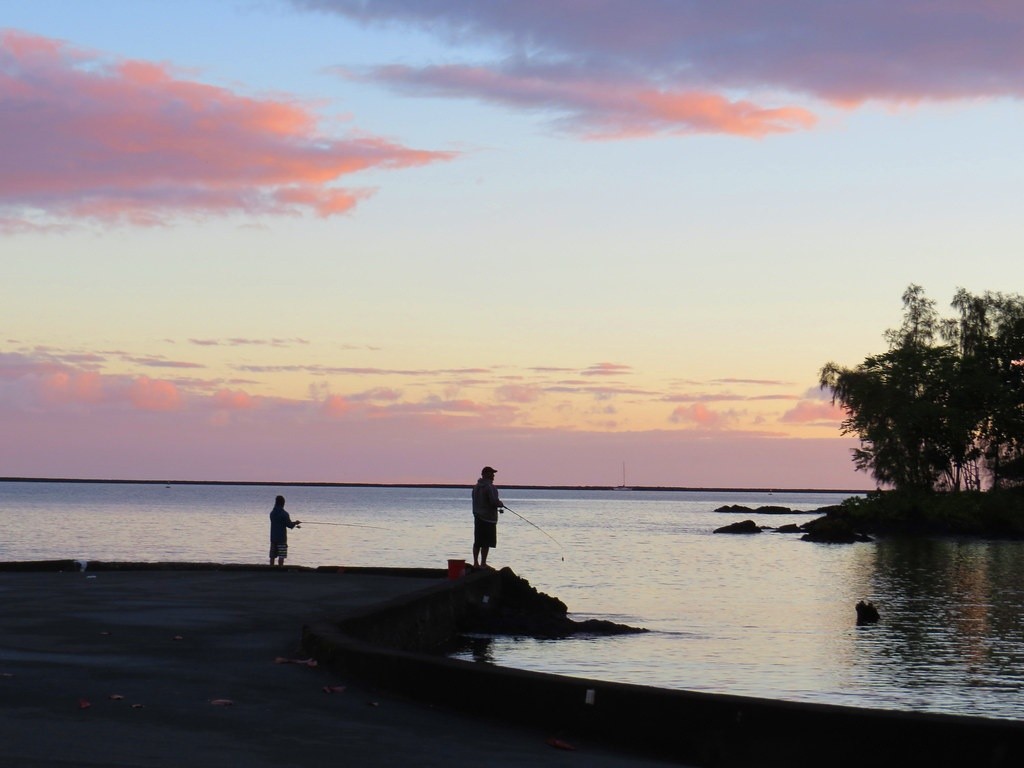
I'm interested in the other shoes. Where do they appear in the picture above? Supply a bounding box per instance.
[479,564,495,571]
[473,564,480,570]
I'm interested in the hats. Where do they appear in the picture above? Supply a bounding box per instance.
[482,467,498,475]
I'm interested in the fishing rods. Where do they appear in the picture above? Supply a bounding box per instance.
[296,521,395,532]
[503,504,566,563]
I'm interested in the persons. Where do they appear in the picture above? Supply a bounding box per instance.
[472,466,504,570]
[270,496,301,566]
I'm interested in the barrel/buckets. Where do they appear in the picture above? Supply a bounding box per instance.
[448,560,466,581]
